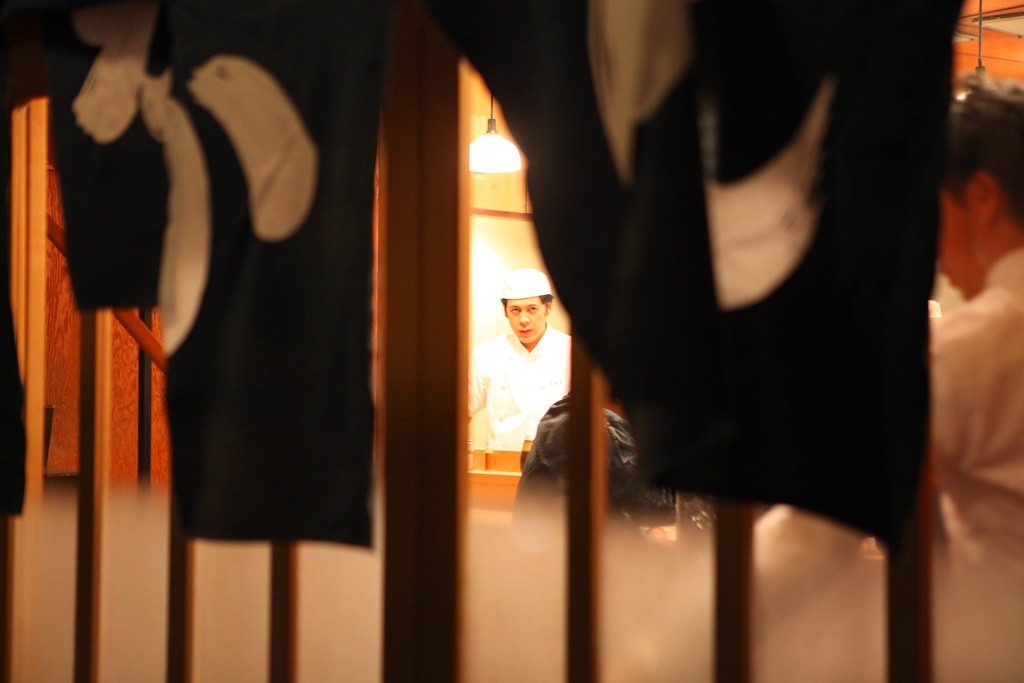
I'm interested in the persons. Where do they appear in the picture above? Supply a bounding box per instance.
[513,365,665,541]
[466,269,571,453]
[748,78,1022,682]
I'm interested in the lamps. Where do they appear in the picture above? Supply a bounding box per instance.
[956,0,987,101]
[469,92,523,174]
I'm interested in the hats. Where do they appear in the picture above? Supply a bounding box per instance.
[500,269,552,300]
